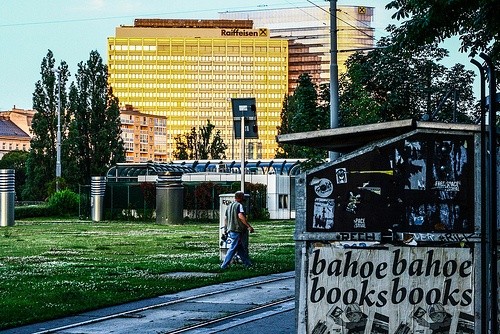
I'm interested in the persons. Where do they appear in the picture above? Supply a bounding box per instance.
[218,190,259,270]
